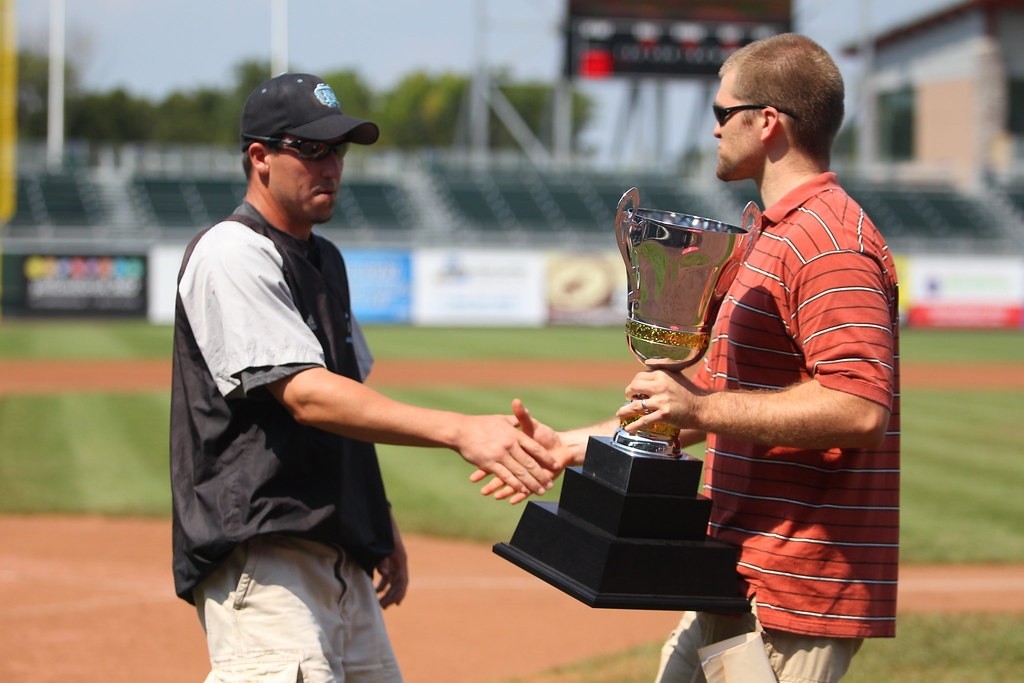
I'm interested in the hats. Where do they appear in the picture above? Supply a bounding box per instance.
[240,74,379,153]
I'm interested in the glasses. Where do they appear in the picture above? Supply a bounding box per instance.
[240,133,347,160]
[713,105,800,126]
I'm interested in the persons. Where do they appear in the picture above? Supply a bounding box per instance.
[471,34,900,683]
[169,73,560,683]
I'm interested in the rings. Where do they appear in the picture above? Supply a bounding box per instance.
[641,399,649,411]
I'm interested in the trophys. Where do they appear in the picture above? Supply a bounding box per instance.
[489,185,761,612]
